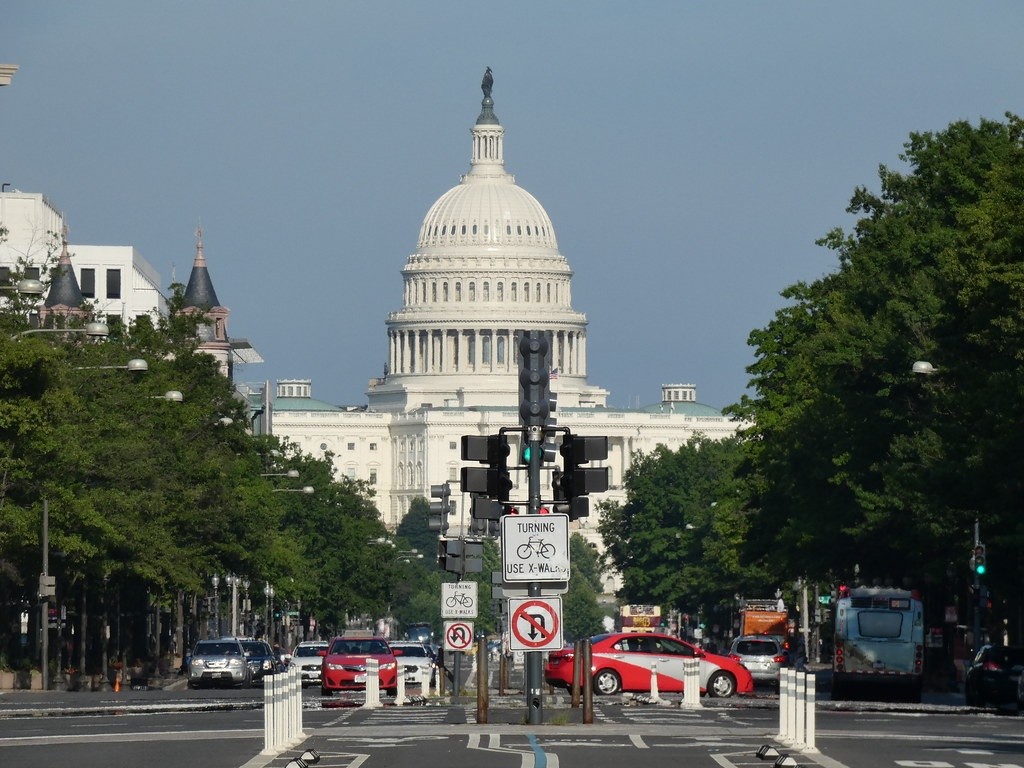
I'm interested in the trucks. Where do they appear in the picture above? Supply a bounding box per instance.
[737,597,791,646]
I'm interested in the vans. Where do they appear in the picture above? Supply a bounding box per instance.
[832,586,926,700]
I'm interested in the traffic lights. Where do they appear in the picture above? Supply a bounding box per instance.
[518,446,545,468]
[550,471,580,523]
[461,432,511,519]
[545,391,559,462]
[428,484,456,534]
[560,433,609,523]
[438,539,464,576]
[974,544,987,575]
[494,602,507,614]
[489,572,504,600]
[503,507,519,517]
[539,508,550,516]
[661,617,666,627]
[495,618,509,633]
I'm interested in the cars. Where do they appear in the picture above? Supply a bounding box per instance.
[542,632,754,699]
[185,639,253,689]
[235,640,280,684]
[318,636,404,695]
[389,640,436,688]
[964,643,1024,710]
[284,641,330,690]
[726,636,788,683]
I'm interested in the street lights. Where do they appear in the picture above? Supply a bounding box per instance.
[39,357,149,691]
[211,573,278,651]
